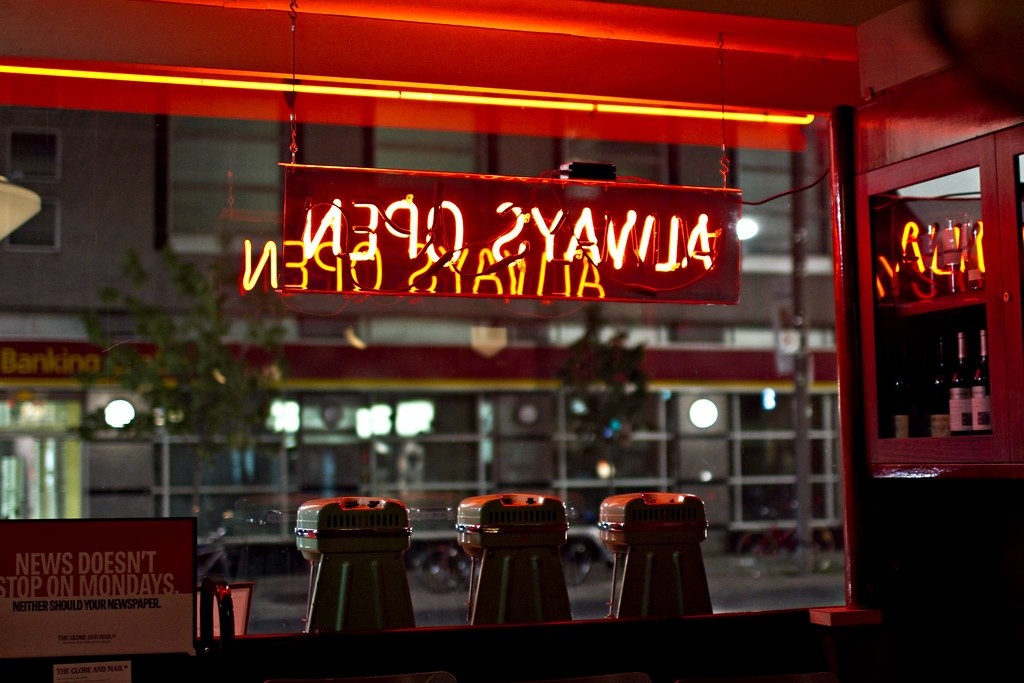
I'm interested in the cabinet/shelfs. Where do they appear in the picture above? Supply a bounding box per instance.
[854,40,1023,477]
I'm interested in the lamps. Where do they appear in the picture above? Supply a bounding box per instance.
[0,175,42,240]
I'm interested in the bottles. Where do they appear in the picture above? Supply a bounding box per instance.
[883,329,992,438]
[918,216,984,299]
